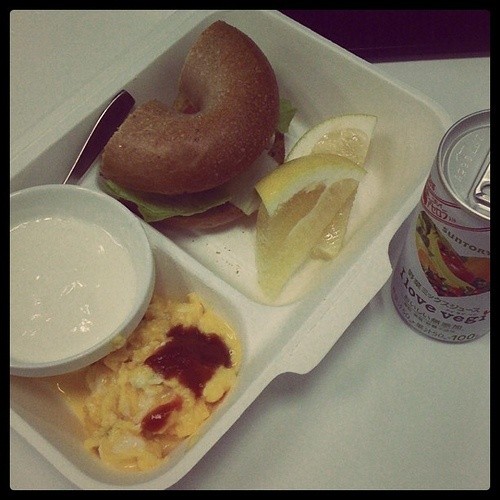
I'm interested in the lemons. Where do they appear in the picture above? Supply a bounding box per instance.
[284,111,379,258]
[252,154,366,299]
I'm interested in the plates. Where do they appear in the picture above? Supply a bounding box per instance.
[9,11,452,493]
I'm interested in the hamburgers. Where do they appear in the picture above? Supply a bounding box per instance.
[97,16,298,236]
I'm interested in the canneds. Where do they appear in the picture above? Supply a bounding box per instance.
[392,106,490,343]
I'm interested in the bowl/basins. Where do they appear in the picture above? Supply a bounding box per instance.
[8,184,156,381]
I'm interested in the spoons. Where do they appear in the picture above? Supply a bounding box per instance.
[62,90,136,187]
